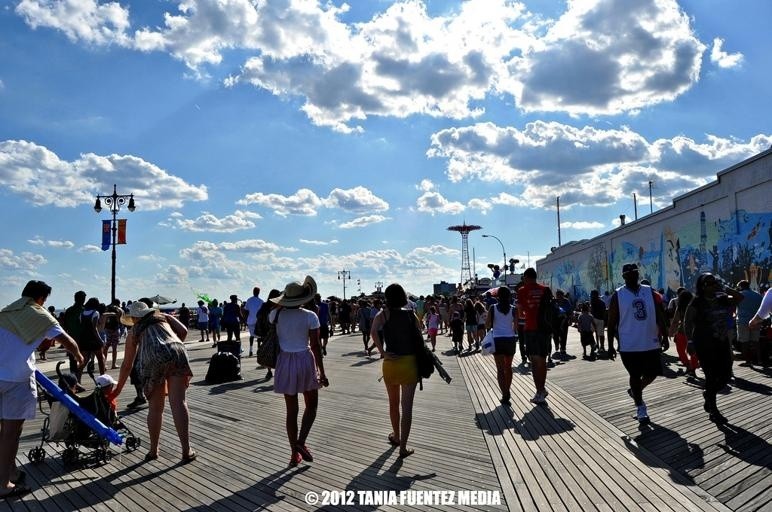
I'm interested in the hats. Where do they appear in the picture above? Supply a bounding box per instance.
[59,373,78,389]
[96,374,118,387]
[269,276,317,307]
[120,302,160,327]
[623,264,641,274]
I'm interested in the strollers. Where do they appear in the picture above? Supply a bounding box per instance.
[32,369,119,469]
[54,359,143,450]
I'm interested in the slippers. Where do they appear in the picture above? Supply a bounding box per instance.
[0,483,31,498]
[182,450,198,461]
[146,452,157,459]
[10,470,26,483]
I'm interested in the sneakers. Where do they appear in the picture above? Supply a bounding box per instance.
[502,392,510,404]
[627,390,649,422]
[703,390,728,423]
[530,388,547,403]
[127,397,146,407]
[291,440,313,464]
[389,432,401,445]
[400,449,414,458]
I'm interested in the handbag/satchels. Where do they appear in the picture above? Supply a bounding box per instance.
[539,287,557,332]
[417,347,435,378]
[257,325,279,368]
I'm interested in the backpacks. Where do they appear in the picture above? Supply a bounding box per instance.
[383,310,423,355]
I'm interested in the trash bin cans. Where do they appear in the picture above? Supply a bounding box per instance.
[212,340,241,367]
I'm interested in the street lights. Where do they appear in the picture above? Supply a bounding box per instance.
[482,232,507,288]
[555,195,561,246]
[648,180,653,214]
[335,267,352,302]
[95,185,135,301]
[375,281,384,292]
